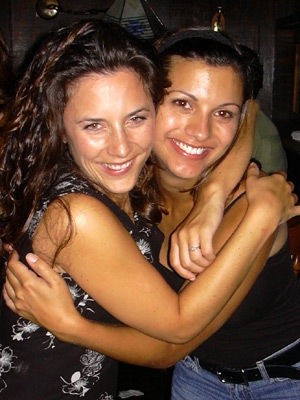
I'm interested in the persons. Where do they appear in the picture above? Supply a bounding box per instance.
[0,19,300,400]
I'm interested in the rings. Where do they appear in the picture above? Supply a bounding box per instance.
[189,246,200,252]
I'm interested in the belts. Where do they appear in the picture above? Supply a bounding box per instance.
[189,342,300,383]
